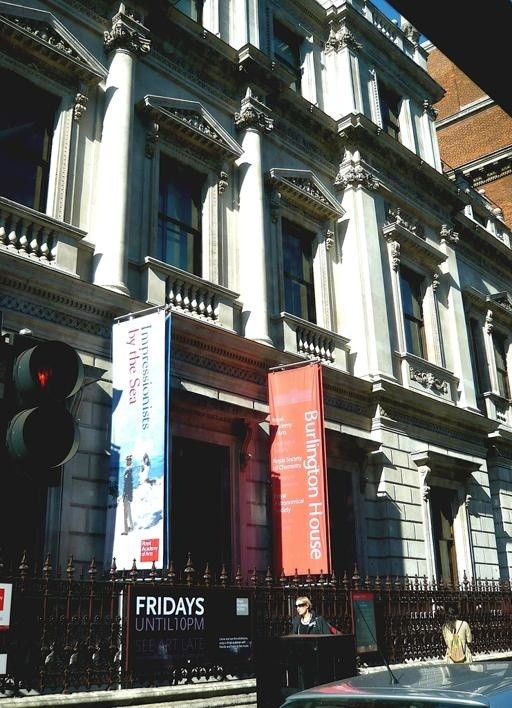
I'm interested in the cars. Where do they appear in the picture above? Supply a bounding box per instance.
[283,599,512,707]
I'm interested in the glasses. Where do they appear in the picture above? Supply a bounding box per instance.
[295,603,306,608]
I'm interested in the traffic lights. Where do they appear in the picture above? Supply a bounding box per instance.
[0,327,86,487]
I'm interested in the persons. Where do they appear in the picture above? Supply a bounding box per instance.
[132,452,164,530]
[290,593,335,635]
[439,602,474,665]
[120,455,135,536]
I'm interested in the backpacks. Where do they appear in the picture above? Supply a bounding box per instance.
[444,620,466,663]
